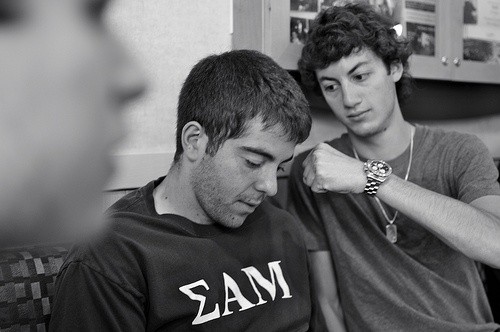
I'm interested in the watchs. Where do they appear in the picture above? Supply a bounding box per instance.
[362,160,392,197]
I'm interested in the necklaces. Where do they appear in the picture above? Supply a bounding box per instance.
[352,122,413,245]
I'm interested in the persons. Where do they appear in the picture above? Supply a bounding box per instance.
[0,0,145,248]
[47,50,309,332]
[288,2,500,332]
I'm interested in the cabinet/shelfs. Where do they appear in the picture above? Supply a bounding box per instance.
[232,0,500,85]
